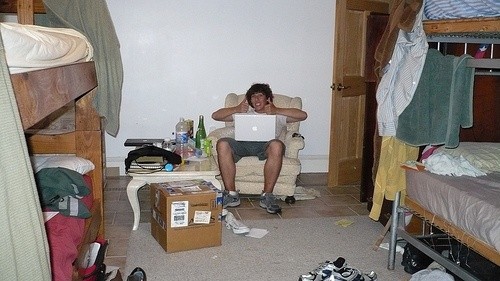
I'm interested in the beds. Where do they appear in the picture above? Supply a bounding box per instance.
[0,1,122,281]
[388,0,500,281]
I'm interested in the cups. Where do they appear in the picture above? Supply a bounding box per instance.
[200,138,213,158]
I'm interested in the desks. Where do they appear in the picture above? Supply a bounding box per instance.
[127,149,221,230]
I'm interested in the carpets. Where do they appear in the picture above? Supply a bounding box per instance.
[126,214,416,281]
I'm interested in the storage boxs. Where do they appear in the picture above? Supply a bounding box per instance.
[150,179,224,254]
[234,112,285,143]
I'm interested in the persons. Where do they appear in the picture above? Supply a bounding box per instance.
[211,84,307,214]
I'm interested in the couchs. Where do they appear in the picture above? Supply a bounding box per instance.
[205,93,305,203]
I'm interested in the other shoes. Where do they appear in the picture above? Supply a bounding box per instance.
[400,238,437,274]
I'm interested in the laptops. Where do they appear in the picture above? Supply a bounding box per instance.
[234,114,276,142]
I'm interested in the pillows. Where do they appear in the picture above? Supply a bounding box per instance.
[418,142,499,178]
[29,155,97,178]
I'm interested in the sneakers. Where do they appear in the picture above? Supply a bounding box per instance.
[219,188,241,209]
[298,256,367,281]
[259,190,282,213]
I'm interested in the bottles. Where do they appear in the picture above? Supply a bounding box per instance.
[175,117,189,160]
[195,115,206,150]
